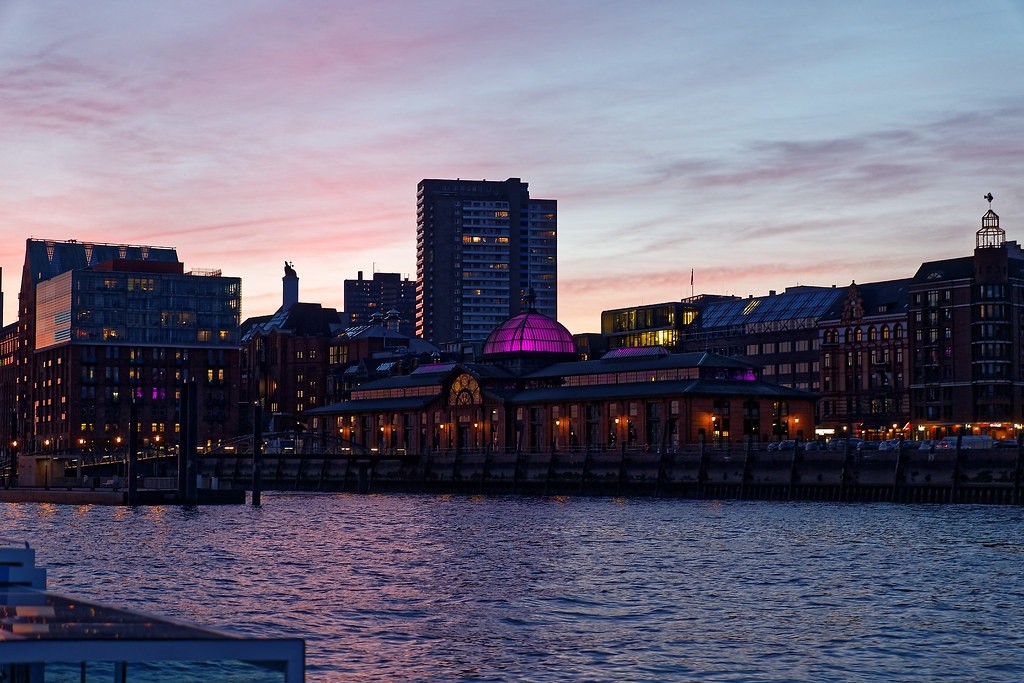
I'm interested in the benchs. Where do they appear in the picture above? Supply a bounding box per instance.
[102,480,114,487]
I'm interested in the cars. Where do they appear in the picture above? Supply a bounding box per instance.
[895,440,921,450]
[768,440,826,451]
[994,440,1024,449]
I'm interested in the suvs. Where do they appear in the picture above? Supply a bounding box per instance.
[836,438,862,450]
[878,440,898,450]
[936,439,972,450]
[828,439,838,450]
[856,441,879,450]
[919,440,939,449]
